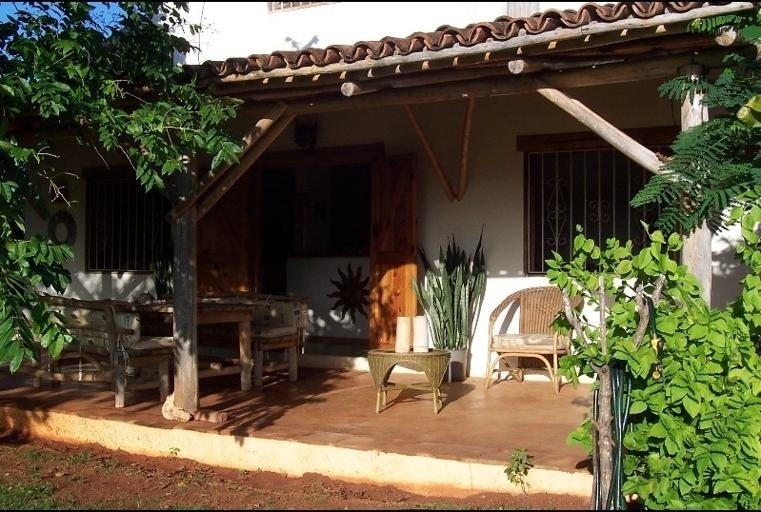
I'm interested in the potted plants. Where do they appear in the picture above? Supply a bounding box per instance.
[411,224,486,384]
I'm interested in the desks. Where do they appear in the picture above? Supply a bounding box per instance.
[93,300,272,402]
[368,348,451,414]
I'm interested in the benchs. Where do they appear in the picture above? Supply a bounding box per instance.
[196,291,309,387]
[33,295,174,408]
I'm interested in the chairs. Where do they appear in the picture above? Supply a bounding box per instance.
[485,286,583,396]
